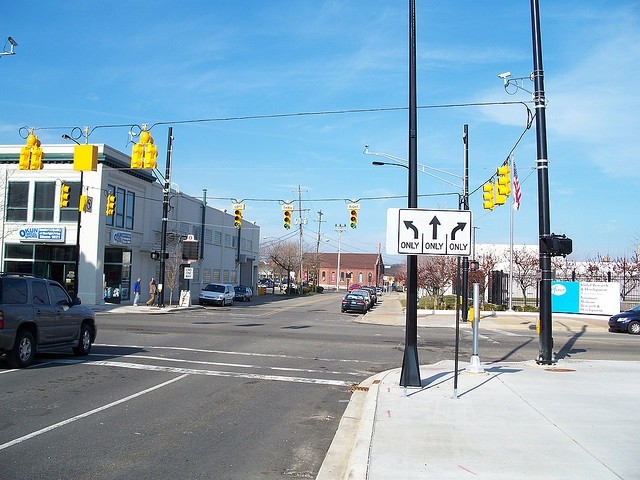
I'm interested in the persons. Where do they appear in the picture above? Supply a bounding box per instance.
[146,278,157,307]
[133,277,141,306]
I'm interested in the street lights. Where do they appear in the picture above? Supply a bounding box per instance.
[372,161,421,388]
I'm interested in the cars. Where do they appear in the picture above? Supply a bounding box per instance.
[342,294,366,313]
[365,286,377,301]
[608,302,640,335]
[350,290,371,307]
[349,284,361,289]
[234,286,252,302]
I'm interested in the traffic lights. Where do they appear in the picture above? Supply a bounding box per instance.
[60,185,71,208]
[482,183,495,210]
[107,196,116,215]
[497,163,510,195]
[350,210,358,228]
[284,211,291,228]
[234,209,242,228]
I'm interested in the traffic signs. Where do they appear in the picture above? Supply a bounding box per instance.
[397,208,473,254]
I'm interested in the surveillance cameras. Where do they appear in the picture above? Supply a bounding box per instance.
[7,36,19,47]
[364,143,370,148]
[498,71,511,79]
[128,131,137,137]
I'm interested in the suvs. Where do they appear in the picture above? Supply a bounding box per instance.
[199,283,235,306]
[0,272,96,367]
